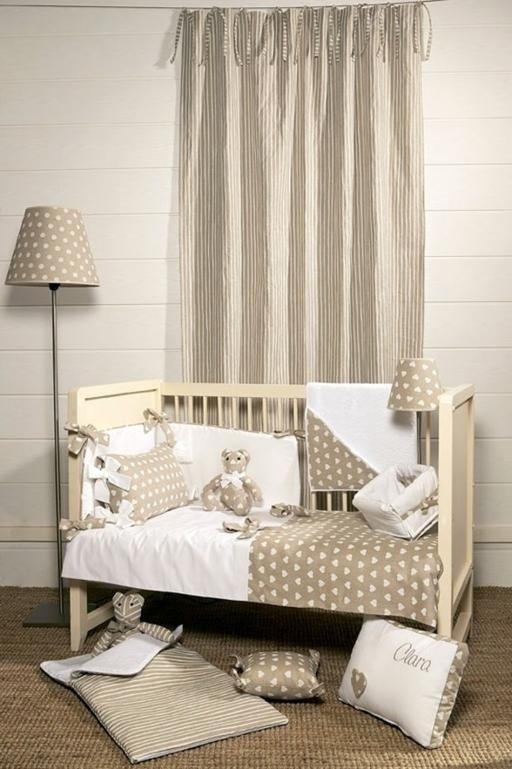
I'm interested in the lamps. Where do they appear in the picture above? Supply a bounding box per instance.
[4,205,102,630]
[387,358,445,464]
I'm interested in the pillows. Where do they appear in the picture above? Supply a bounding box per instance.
[229,649,325,701]
[337,615,470,750]
[98,440,198,529]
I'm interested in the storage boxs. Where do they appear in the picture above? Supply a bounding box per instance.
[352,464,438,540]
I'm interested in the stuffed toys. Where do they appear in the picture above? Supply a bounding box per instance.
[90,590,175,657]
[201,447,264,516]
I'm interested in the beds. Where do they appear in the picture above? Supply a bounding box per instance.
[70,378,475,651]
